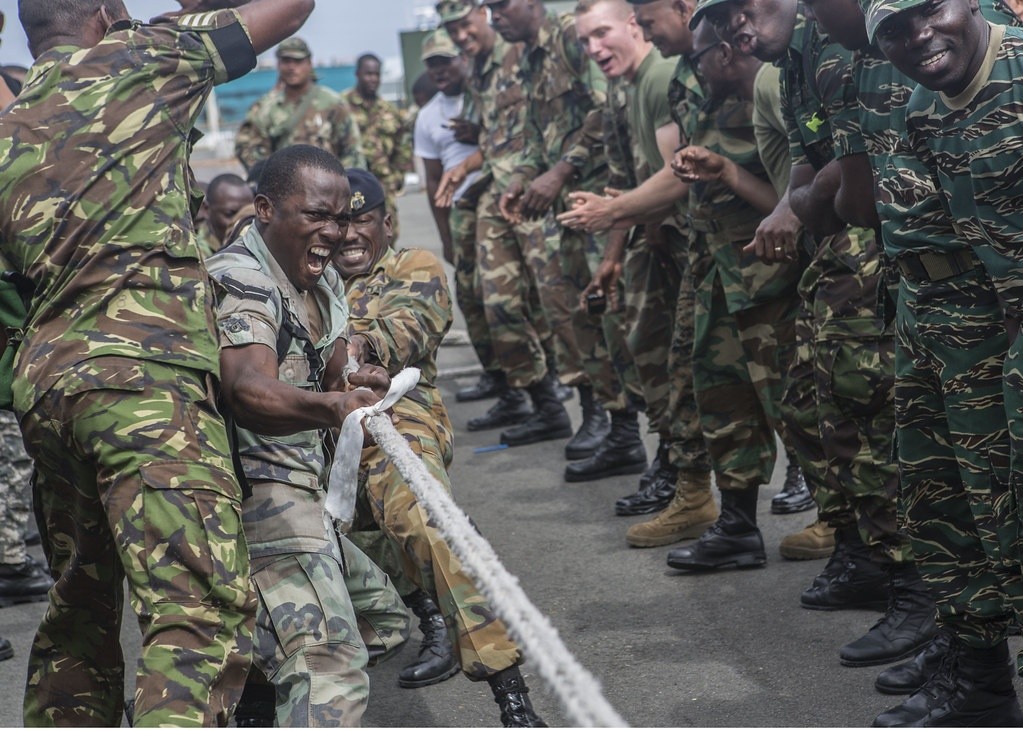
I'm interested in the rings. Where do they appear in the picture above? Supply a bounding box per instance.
[775,246,783,251]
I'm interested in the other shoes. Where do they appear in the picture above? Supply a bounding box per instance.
[234,672,277,728]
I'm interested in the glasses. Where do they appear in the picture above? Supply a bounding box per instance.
[688,40,724,66]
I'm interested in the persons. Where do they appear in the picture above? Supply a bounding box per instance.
[412,0,1023,728]
[0,0,602,728]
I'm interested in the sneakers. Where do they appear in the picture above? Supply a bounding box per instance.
[0,638,14,661]
[0,554,56,607]
[22,531,40,545]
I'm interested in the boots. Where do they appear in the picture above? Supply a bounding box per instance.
[625,471,717,546]
[500,375,573,445]
[771,453,816,512]
[565,408,647,481]
[779,517,837,561]
[799,528,1023,728]
[565,386,611,461]
[615,448,677,517]
[398,597,461,688]
[640,448,666,491]
[488,666,548,728]
[457,370,509,402]
[467,385,533,431]
[667,485,766,569]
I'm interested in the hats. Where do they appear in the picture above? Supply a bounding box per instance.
[420,30,462,61]
[275,38,310,60]
[859,0,926,46]
[689,0,724,31]
[436,0,478,28]
[345,168,386,215]
